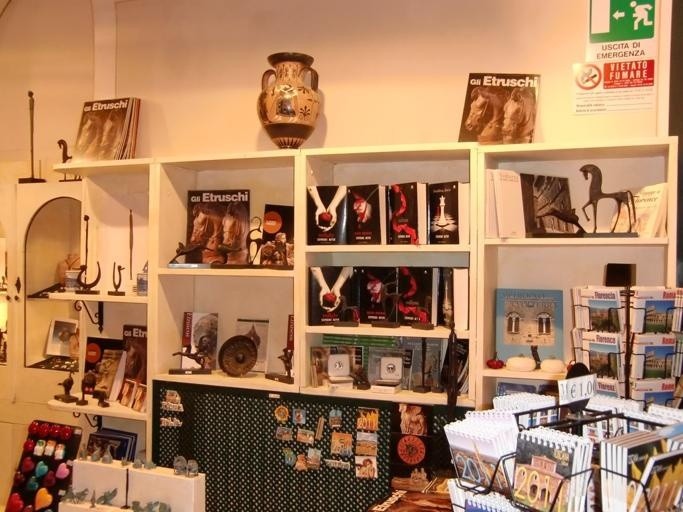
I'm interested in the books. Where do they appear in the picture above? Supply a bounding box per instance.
[72,98,141,161]
[455,72,545,145]
[44,316,148,462]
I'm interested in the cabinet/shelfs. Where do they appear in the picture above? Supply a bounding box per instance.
[46,135,677,464]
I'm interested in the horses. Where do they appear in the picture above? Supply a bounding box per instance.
[122,337,146,384]
[76,110,122,160]
[463,85,536,145]
[189,198,248,266]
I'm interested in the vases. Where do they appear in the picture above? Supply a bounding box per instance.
[255,52,321,150]
